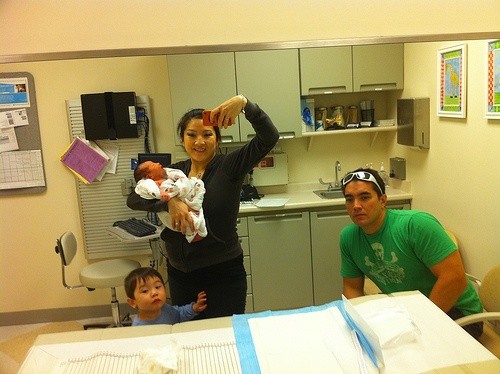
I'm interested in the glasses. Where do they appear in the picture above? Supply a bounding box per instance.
[343,171,382,195]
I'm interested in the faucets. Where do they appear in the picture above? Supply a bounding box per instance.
[334,161,341,188]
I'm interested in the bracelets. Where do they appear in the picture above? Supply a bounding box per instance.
[240,94,246,114]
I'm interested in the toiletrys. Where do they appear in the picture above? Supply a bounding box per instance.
[379,162,387,186]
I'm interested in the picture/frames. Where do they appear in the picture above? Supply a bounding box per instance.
[484,38,500,120]
[436,43,467,119]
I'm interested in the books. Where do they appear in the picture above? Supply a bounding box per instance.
[59,137,119,185]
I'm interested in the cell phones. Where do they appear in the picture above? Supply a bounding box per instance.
[202,110,232,126]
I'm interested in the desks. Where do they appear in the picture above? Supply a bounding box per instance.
[105,222,171,299]
[18,315,242,373]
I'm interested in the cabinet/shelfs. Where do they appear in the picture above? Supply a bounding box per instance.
[235,204,411,312]
[165,42,407,150]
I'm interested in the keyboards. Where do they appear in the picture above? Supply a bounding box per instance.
[119,217,157,237]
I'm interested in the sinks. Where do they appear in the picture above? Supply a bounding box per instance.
[312,189,345,200]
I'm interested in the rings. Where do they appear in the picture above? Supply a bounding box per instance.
[175,222,180,225]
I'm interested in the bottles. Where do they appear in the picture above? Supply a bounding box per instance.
[316,106,329,130]
[347,105,360,124]
[331,105,346,128]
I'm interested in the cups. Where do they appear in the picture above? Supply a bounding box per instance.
[361,109,374,127]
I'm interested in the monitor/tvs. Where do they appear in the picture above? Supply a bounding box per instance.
[138,153,170,168]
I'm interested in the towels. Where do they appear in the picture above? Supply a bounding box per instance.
[134,167,208,243]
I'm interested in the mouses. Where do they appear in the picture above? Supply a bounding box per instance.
[113,221,122,227]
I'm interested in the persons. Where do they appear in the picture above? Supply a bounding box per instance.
[134,160,208,244]
[123,92,281,325]
[337,168,484,340]
[123,267,207,327]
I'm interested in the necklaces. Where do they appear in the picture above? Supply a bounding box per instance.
[190,165,206,180]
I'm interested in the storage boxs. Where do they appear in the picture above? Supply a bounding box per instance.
[301,99,315,132]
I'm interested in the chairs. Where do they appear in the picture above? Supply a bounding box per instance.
[454,265,500,362]
[55,232,141,328]
